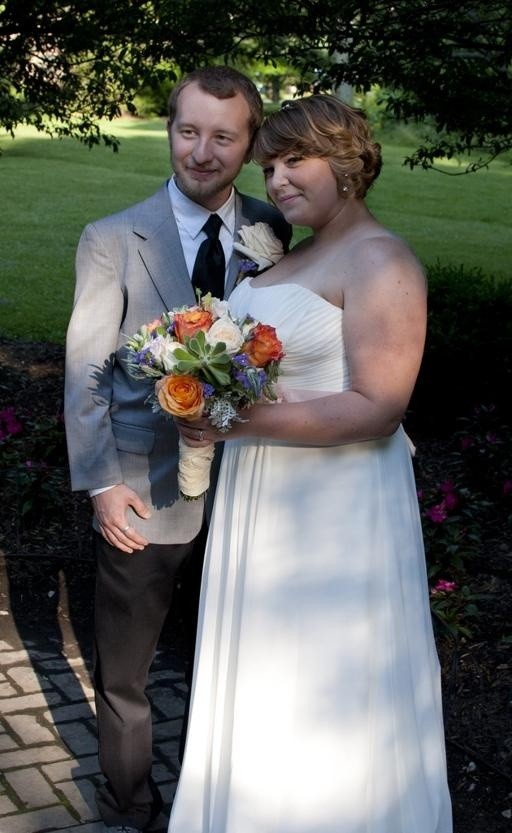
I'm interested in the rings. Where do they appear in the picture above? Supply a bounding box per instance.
[122,526,131,533]
[198,430,204,440]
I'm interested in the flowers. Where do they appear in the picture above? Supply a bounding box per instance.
[114,288,284,504]
[235,221,286,286]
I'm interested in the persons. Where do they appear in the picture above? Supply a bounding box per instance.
[60,63,293,828]
[161,92,455,828]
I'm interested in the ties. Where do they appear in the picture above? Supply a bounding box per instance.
[192,213,224,301]
